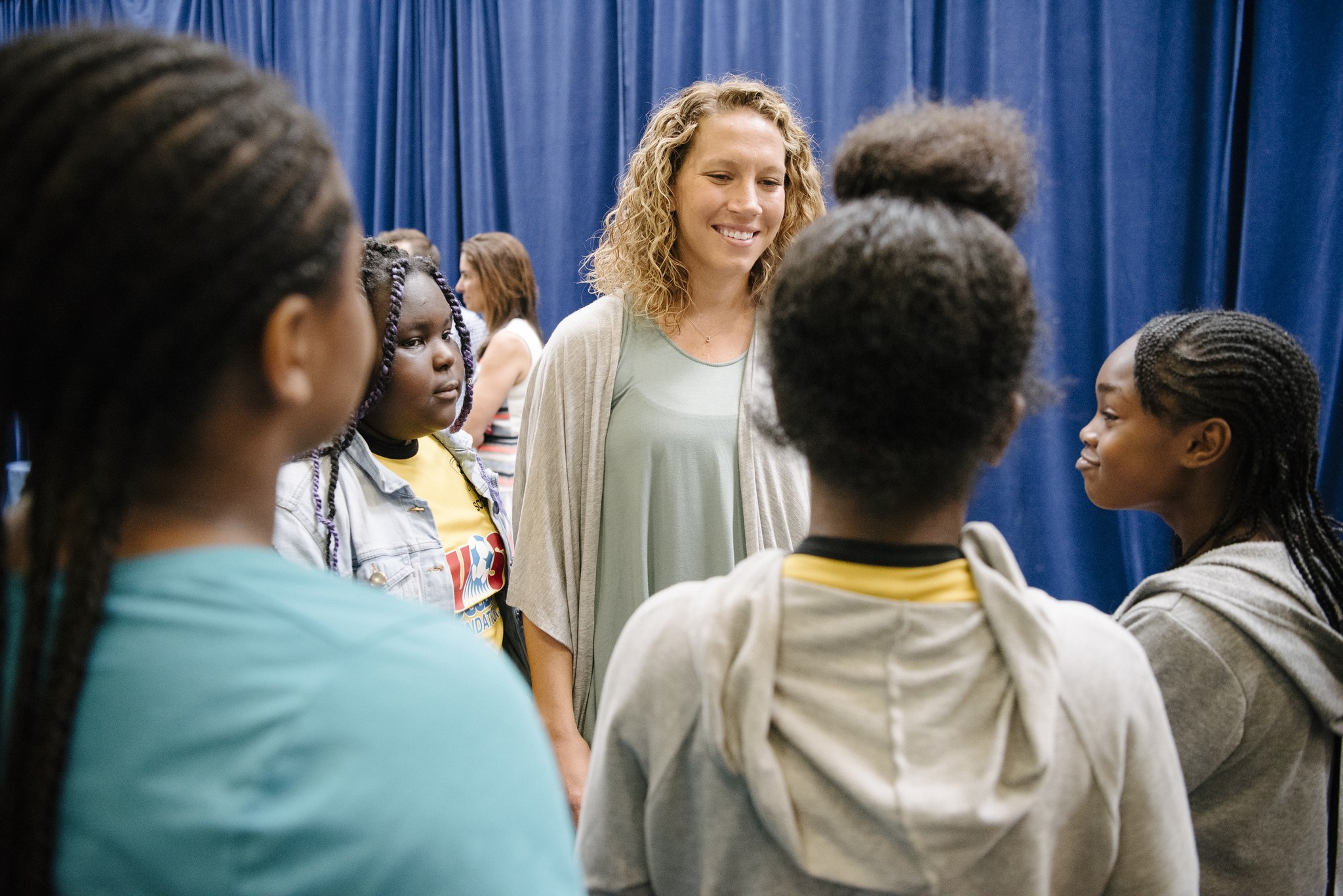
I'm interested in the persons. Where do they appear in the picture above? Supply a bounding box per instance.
[572,88,1202,896]
[0,22,586,896]
[372,229,547,518]
[272,237,530,687]
[504,73,824,830]
[1070,307,1338,896]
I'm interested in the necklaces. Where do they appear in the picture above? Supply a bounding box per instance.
[669,300,747,340]
